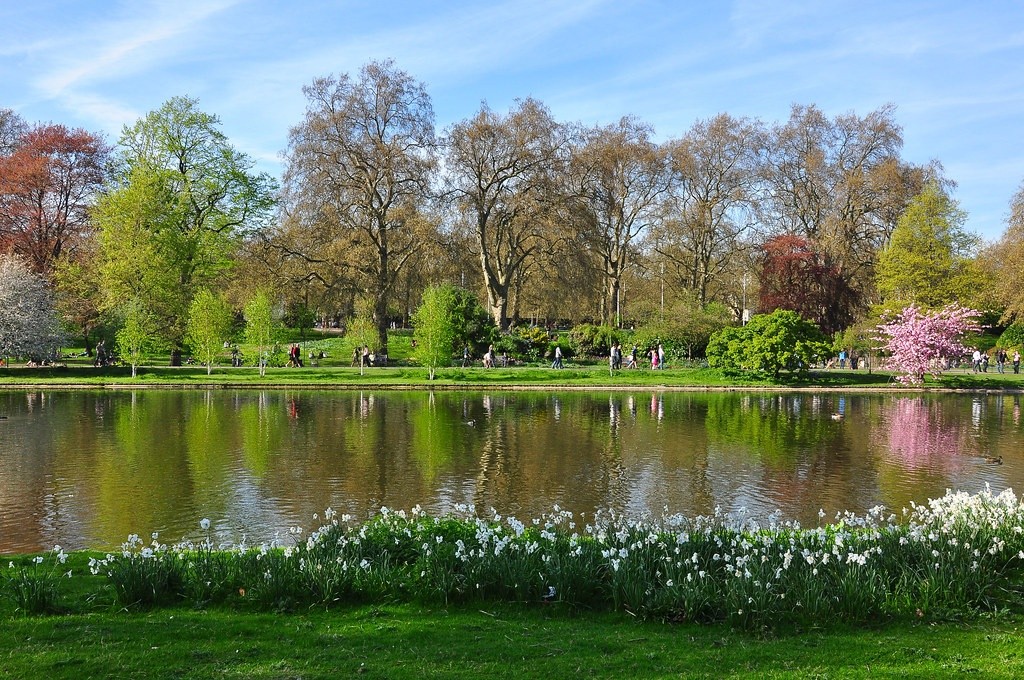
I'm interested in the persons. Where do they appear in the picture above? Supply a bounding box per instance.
[799,348,1021,373]
[185,324,665,370]
